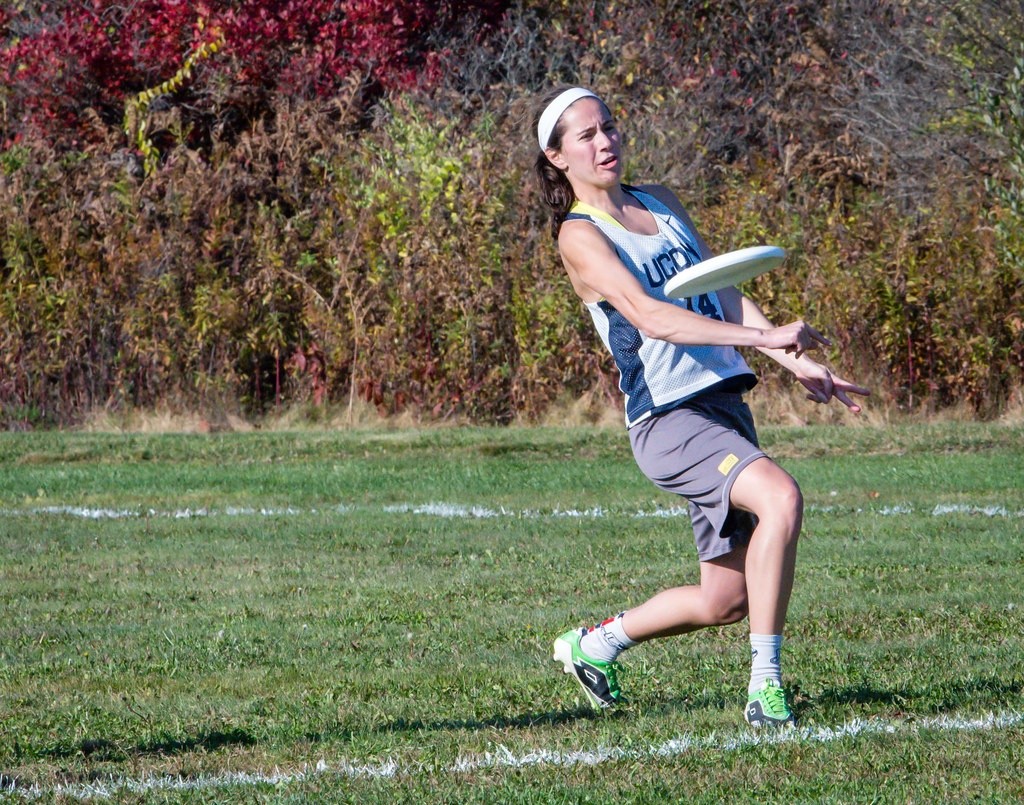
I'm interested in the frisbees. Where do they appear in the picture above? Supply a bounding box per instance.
[663,245,790,301]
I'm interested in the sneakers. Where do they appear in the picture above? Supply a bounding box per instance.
[743,677,795,730]
[553,627,625,717]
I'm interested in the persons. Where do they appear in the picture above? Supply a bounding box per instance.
[529,85,871,733]
